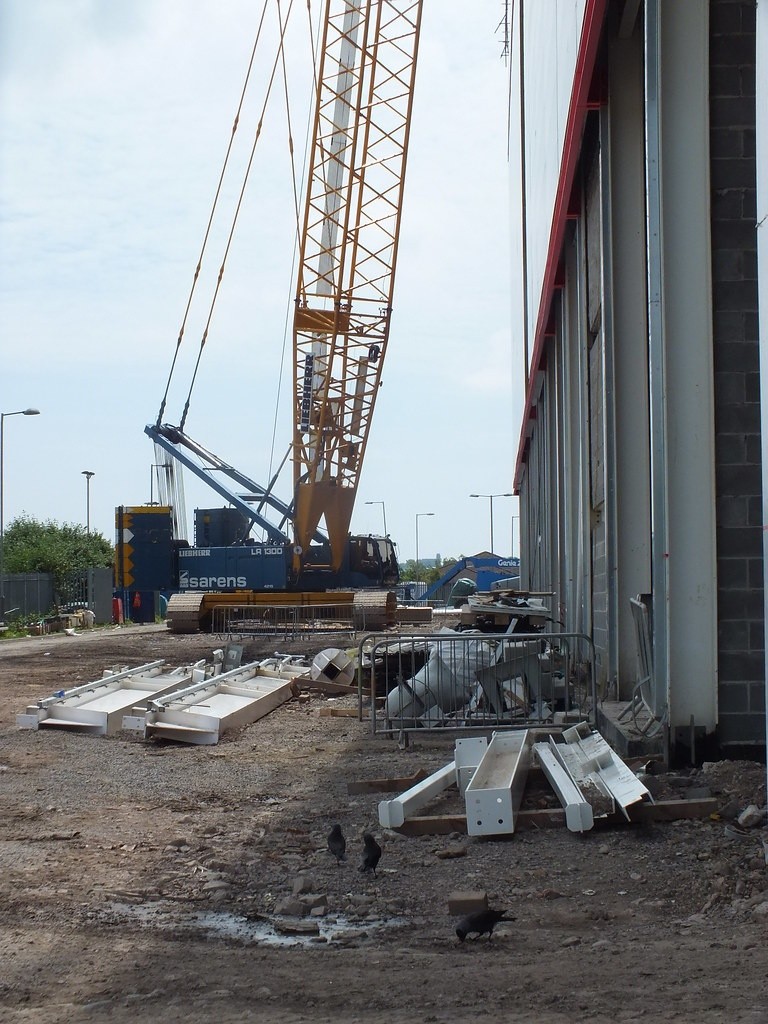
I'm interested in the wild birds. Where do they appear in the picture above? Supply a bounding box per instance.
[325,823,348,867]
[455,909,518,944]
[357,832,382,880]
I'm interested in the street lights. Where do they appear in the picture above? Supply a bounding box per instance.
[469,494,513,554]
[151,464,173,507]
[512,515,520,559]
[416,513,435,607]
[0,408,41,624]
[364,501,386,537]
[81,471,96,534]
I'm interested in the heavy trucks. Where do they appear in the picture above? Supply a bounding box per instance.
[113,422,400,617]
